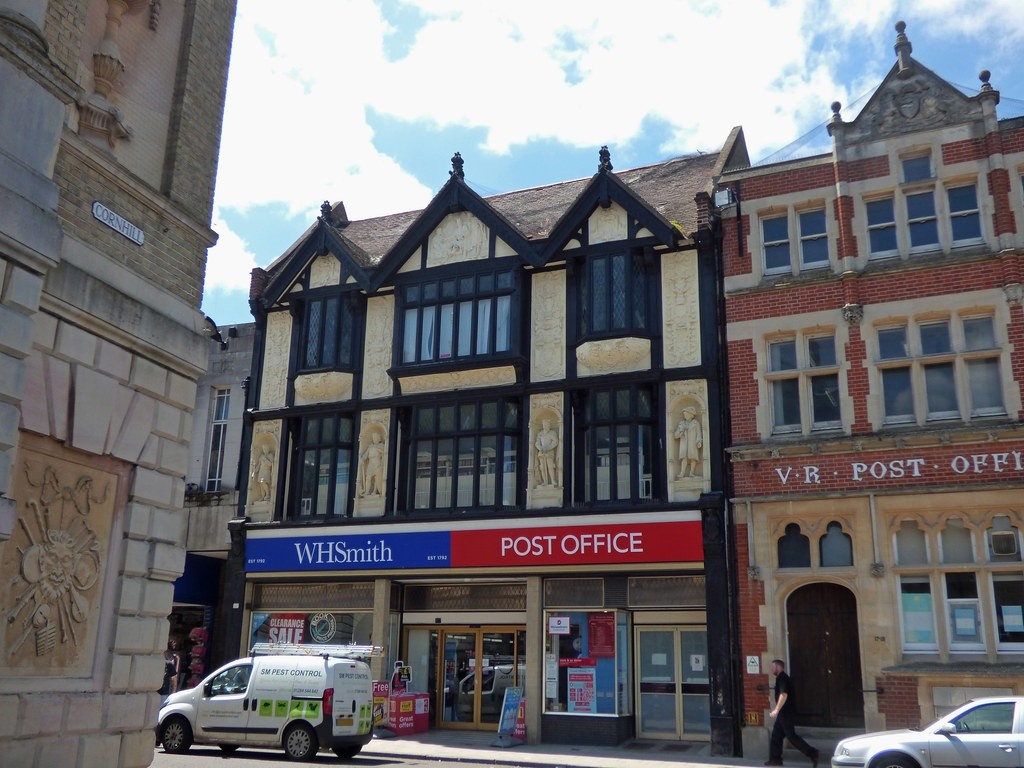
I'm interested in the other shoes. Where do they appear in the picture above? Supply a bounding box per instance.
[764,759,784,765]
[813,750,819,768]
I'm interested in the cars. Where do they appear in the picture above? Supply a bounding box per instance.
[831,695,1024,768]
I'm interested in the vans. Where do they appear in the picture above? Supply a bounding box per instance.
[444,664,525,715]
[157,654,375,762]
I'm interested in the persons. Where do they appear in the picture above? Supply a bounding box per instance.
[764,660,819,768]
[254,443,273,502]
[360,431,385,497]
[534,419,558,486]
[155,638,180,748]
[673,406,702,478]
